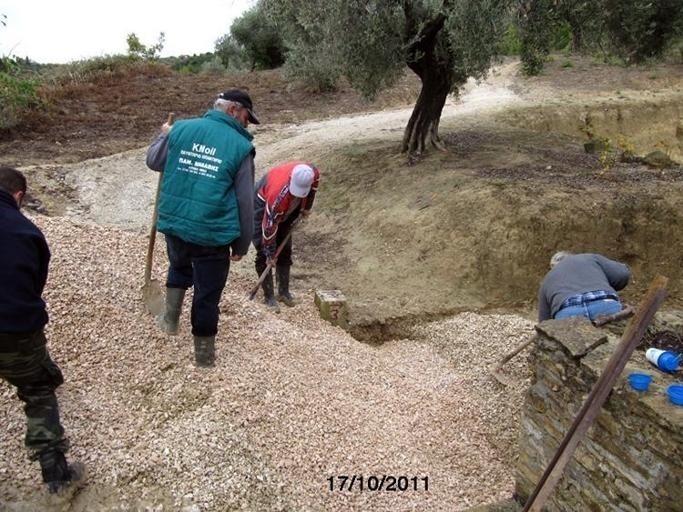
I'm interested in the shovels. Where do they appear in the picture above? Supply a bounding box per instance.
[140,112,175,318]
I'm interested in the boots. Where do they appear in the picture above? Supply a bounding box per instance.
[280,266,297,311]
[32,454,89,511]
[252,268,279,312]
[191,337,219,374]
[155,285,186,341]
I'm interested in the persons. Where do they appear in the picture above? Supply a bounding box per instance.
[145,90,260,367]
[0,166,84,493]
[251,159,320,312]
[538,250,631,325]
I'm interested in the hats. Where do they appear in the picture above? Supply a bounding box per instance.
[286,164,315,197]
[218,89,262,128]
